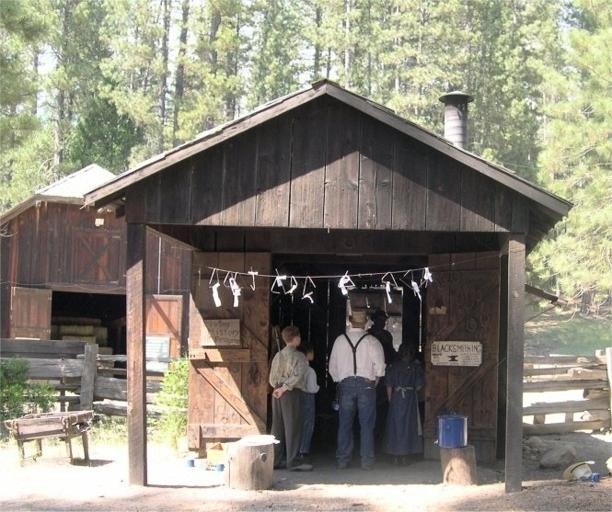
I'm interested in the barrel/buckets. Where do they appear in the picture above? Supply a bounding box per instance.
[434,414,469,449]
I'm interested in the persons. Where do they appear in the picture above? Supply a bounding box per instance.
[269,325,315,473]
[385,343,424,467]
[300,338,320,460]
[327,304,386,471]
[366,308,398,366]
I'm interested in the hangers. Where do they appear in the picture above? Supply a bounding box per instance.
[205,264,433,295]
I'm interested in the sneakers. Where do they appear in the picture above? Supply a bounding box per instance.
[393,455,415,465]
[274,461,312,471]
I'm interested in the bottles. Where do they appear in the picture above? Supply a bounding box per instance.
[181,457,204,491]
[329,400,341,411]
[211,462,228,498]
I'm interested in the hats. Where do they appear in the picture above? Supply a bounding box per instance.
[371,311,388,320]
[348,311,368,324]
